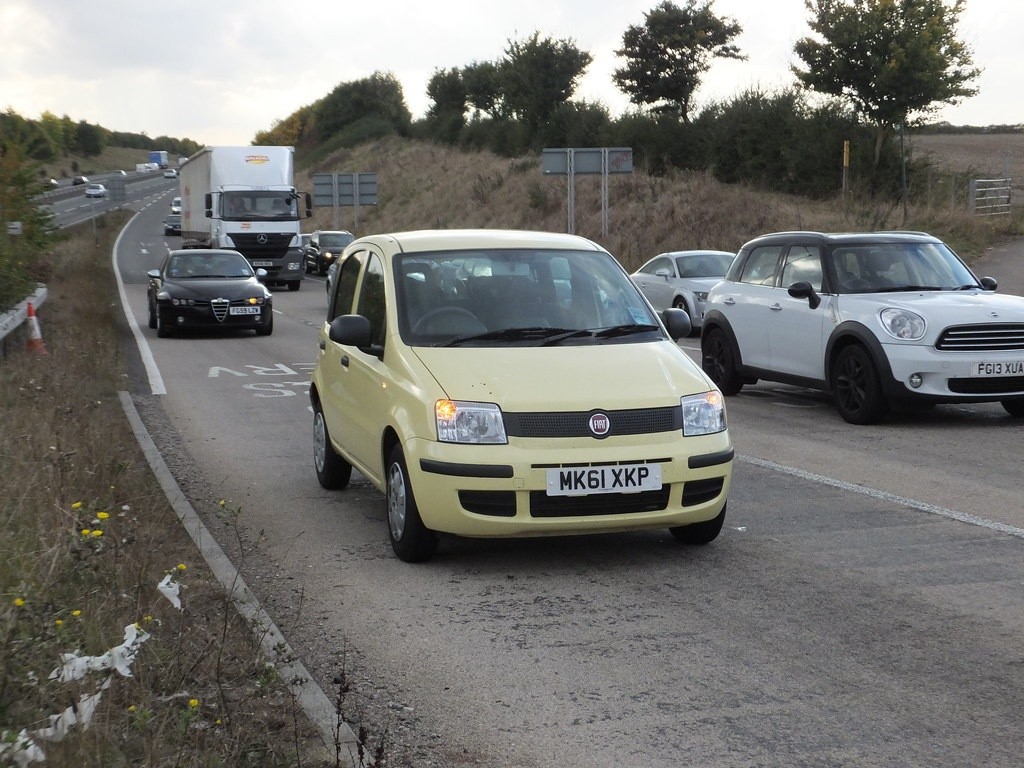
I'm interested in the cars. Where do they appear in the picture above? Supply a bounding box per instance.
[42,178,60,191]
[162,214,181,236]
[619,248,760,339]
[400,255,610,316]
[146,248,276,340]
[113,169,127,177]
[169,197,181,215]
[84,184,107,198]
[72,176,90,187]
[163,168,177,178]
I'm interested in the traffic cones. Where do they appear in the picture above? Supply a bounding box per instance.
[25,301,50,358]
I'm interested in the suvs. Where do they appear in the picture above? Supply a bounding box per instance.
[700,230,1024,424]
[303,229,356,277]
[308,227,735,562]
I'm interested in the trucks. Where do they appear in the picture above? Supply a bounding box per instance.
[134,150,190,174]
[178,146,313,291]
